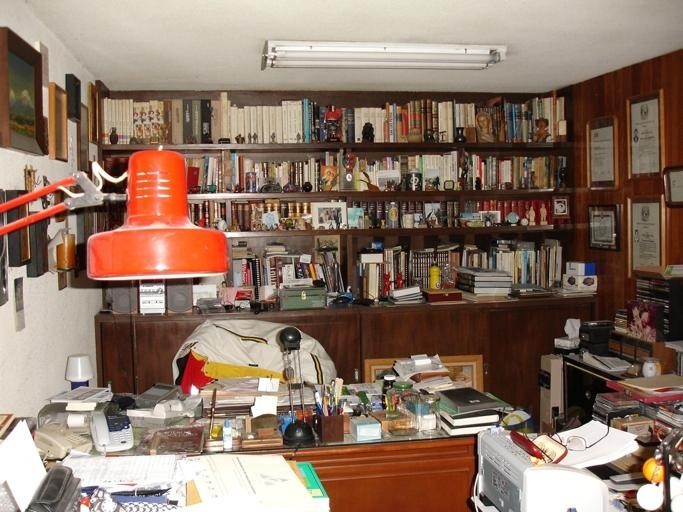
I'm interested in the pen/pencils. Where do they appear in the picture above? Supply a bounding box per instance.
[314,379,347,417]
[209,388,217,439]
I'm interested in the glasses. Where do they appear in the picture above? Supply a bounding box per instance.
[551,415,610,451]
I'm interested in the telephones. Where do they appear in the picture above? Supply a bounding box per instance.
[89,412,134,456]
[33,424,93,459]
[126,399,187,428]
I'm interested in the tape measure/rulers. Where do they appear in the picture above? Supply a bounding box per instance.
[334,377,344,403]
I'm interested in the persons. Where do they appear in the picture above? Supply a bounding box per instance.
[218,282,231,304]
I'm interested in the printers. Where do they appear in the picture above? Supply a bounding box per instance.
[475,426,610,512]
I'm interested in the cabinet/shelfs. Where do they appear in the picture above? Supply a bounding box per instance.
[92,79,601,431]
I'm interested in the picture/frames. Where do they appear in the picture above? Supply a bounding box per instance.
[627,89,665,179]
[626,194,667,278]
[552,195,569,219]
[35,41,49,87]
[364,354,484,393]
[587,204,621,251]
[0,27,43,156]
[662,166,683,208]
[65,73,81,124]
[311,202,348,229]
[88,82,96,144]
[5,190,49,277]
[586,116,619,191]
[77,101,89,173]
[48,82,68,162]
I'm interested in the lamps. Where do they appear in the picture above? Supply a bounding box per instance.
[56,214,76,271]
[87,152,228,281]
[261,40,507,70]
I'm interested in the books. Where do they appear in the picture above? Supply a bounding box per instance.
[228,99,567,232]
[233,241,567,304]
[49,385,110,412]
[590,391,683,476]
[431,388,500,438]
[106,278,194,314]
[101,97,226,231]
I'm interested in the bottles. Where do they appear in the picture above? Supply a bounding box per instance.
[385,381,419,417]
[382,375,396,409]
[386,201,399,228]
[429,260,442,290]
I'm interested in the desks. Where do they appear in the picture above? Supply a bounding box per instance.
[205,405,478,511]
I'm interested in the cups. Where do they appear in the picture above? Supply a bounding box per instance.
[417,393,441,436]
